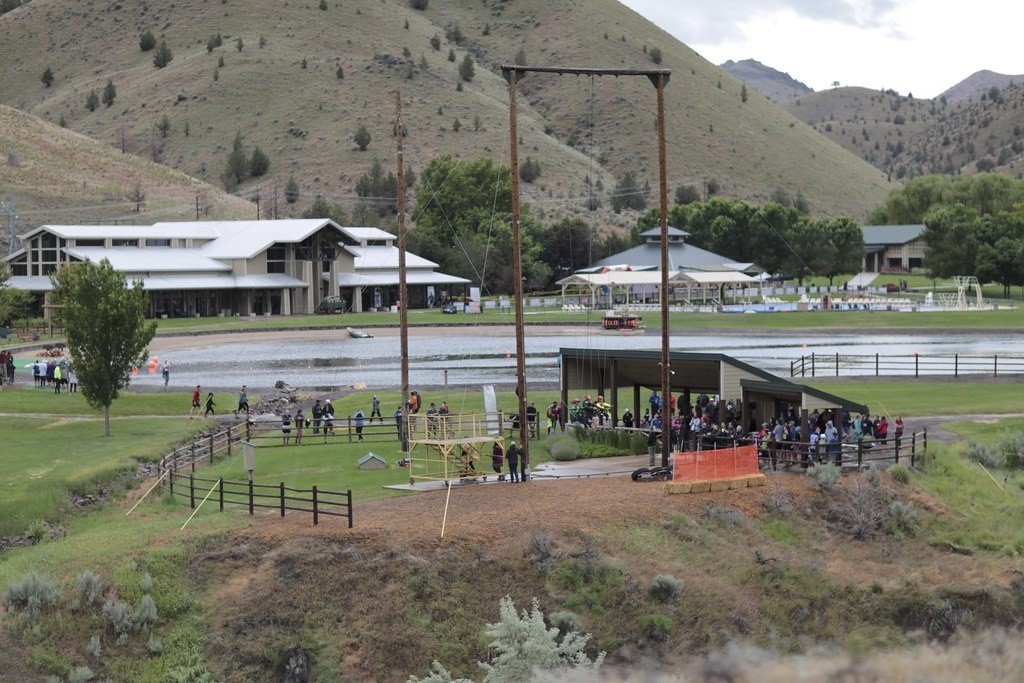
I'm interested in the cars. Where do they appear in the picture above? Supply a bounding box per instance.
[443,305,457,314]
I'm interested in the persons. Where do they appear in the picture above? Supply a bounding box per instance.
[367,395,383,424]
[189,385,204,419]
[354,408,365,443]
[282,410,292,447]
[33,356,77,394]
[505,441,522,483]
[623,390,904,468]
[492,437,505,480]
[204,392,217,418]
[159,359,172,386]
[426,402,448,439]
[505,401,536,439]
[232,385,251,419]
[408,390,421,414]
[0,349,16,385]
[546,395,614,434]
[394,406,402,439]
[312,399,335,444]
[294,410,304,446]
[461,436,475,473]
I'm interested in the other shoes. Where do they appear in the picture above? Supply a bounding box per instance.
[498,477,505,480]
[516,479,519,483]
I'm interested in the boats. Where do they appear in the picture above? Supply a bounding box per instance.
[602,315,643,330]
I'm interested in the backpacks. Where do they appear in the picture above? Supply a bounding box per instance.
[548,407,555,417]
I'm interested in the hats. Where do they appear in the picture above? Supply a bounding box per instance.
[598,396,603,400]
[587,395,591,400]
[762,423,768,427]
[832,432,837,436]
[574,399,581,403]
